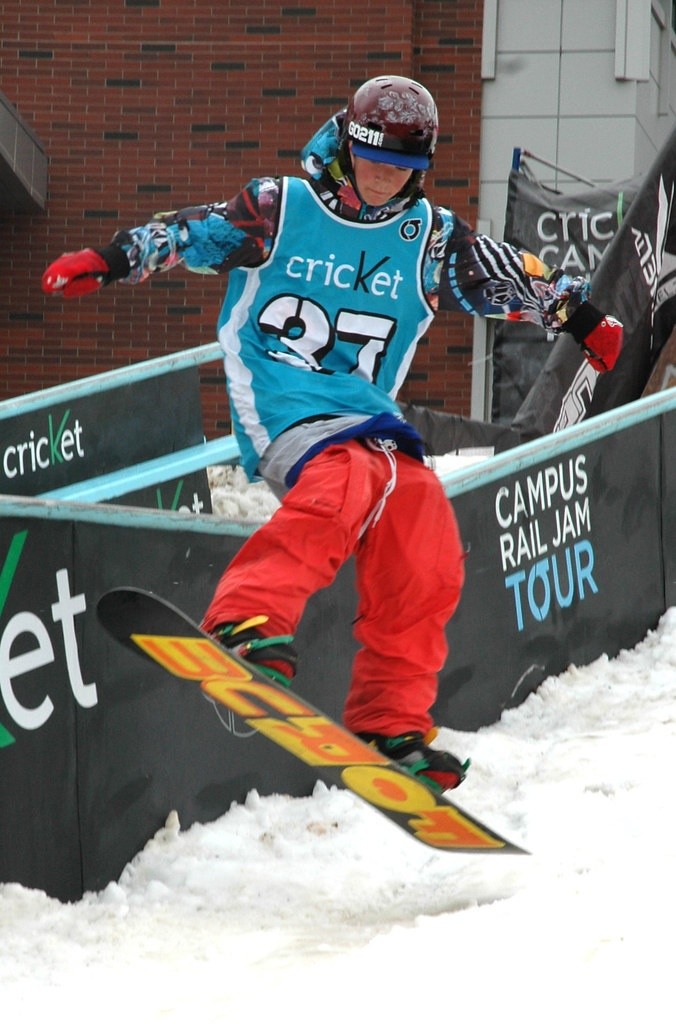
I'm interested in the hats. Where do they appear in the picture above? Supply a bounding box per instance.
[351,142,430,170]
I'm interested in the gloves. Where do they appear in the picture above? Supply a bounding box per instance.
[580,314,623,374]
[41,247,111,299]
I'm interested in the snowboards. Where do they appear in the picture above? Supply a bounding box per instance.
[95,586,535,858]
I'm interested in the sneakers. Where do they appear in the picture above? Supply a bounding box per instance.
[358,727,470,793]
[210,616,297,688]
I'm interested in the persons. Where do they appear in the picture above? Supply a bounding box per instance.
[38,74,626,794]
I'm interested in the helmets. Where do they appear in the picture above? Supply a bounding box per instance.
[341,75,439,183]
